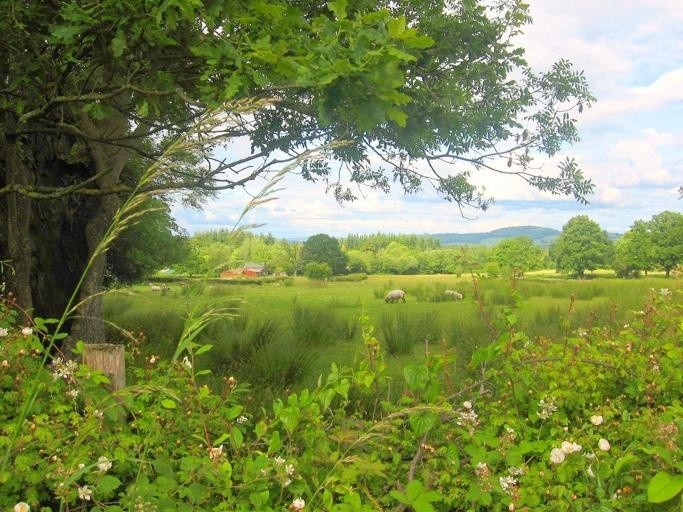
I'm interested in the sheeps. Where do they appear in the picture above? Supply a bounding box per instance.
[384,290,406,303]
[444,290,463,302]
